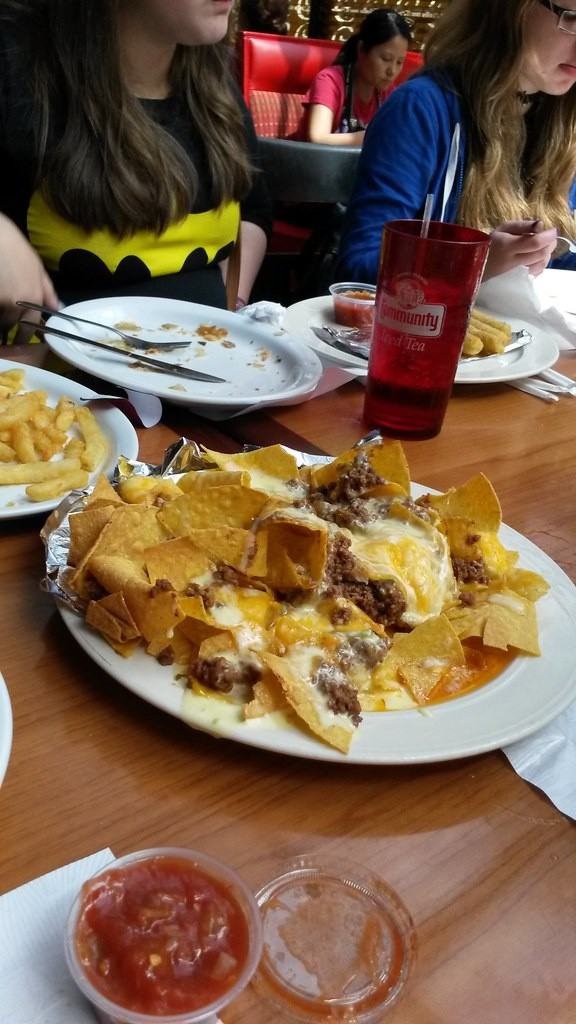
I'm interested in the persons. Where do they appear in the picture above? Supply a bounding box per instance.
[341,1,576,284]
[278,8,412,298]
[0,0,277,346]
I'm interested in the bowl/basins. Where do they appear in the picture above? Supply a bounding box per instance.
[62,847,264,1024]
[328,282,376,324]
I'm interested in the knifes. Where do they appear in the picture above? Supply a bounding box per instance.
[18,317,227,384]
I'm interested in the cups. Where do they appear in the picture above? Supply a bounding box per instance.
[363,219,492,438]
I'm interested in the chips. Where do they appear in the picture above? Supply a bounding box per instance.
[64,439,551,756]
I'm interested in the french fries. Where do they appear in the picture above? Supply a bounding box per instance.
[0,367,103,502]
[463,309,512,357]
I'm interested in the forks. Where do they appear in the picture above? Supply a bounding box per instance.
[522,231,576,252]
[16,300,206,352]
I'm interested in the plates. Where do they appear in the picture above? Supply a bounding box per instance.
[527,267,576,325]
[286,295,560,384]
[42,296,322,405]
[0,358,139,519]
[55,455,576,764]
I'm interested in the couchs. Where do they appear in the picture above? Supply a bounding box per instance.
[238,32,428,280]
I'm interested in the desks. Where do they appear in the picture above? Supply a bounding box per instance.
[0,343,576,1024]
[258,135,363,203]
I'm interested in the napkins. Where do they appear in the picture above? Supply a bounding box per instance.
[479,265,576,350]
